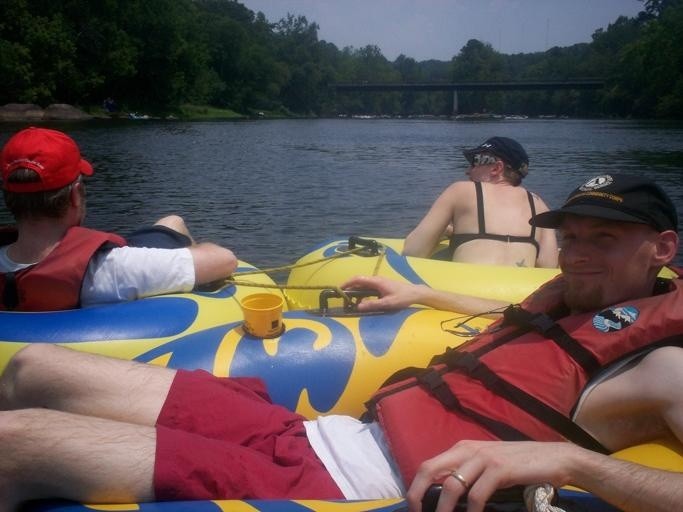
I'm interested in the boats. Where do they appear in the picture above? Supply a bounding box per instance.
[0,255,286,378]
[284,235,678,318]
[57,286,683,511]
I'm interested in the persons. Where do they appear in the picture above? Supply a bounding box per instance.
[1,125,239,310]
[0,170,683,512]
[401,136,560,269]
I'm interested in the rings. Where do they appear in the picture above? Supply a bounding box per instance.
[450,471,469,489]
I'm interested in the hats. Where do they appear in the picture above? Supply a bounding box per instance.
[529,173,679,231]
[0,126,95,194]
[464,136,530,178]
